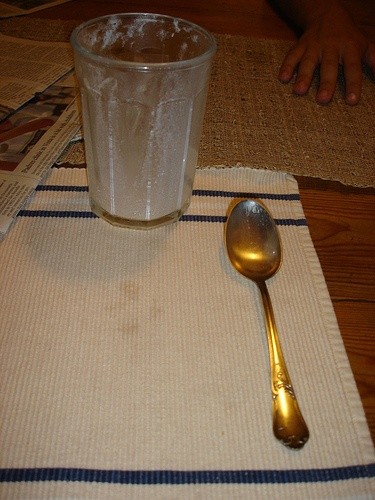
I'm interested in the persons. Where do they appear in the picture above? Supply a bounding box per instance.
[267,1,375,105]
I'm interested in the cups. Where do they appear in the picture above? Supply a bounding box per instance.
[72,13,216,229]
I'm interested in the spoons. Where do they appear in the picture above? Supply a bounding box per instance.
[227,200,310,449]
